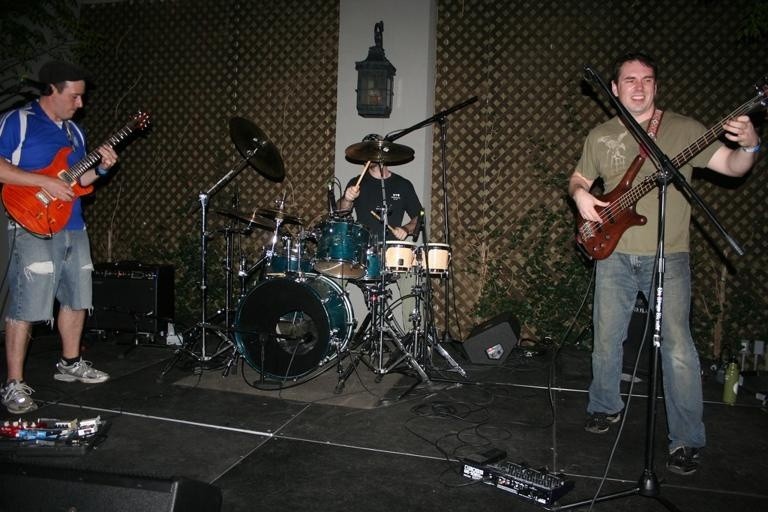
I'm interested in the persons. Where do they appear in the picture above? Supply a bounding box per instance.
[1,59,118,415]
[567,48,759,477]
[330,133,424,335]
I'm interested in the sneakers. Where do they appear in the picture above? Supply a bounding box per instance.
[0,377,38,416]
[51,355,110,384]
[583,411,624,435]
[667,444,702,477]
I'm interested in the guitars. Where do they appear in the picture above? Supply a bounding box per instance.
[1,111,150,238]
[575,84,768,260]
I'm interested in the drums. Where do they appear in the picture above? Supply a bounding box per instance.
[377,240,417,274]
[312,220,370,280]
[417,243,451,278]
[264,255,312,323]
[234,272,355,382]
[356,251,380,281]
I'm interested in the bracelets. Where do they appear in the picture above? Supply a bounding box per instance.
[742,137,762,152]
[94,163,109,177]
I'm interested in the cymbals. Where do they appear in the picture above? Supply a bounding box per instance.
[219,207,274,231]
[258,206,300,223]
[345,141,415,163]
[229,116,285,183]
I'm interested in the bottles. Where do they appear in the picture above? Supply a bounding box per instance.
[721,353,741,407]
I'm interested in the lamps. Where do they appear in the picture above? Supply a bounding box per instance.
[354,21,396,119]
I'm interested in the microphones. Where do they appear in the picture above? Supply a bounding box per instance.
[583,64,595,83]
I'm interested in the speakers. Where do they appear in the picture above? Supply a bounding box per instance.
[0,458,222,512]
[464,312,520,366]
[92,265,175,332]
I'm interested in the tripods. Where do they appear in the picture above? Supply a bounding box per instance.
[160,235,470,396]
[541,178,686,512]
